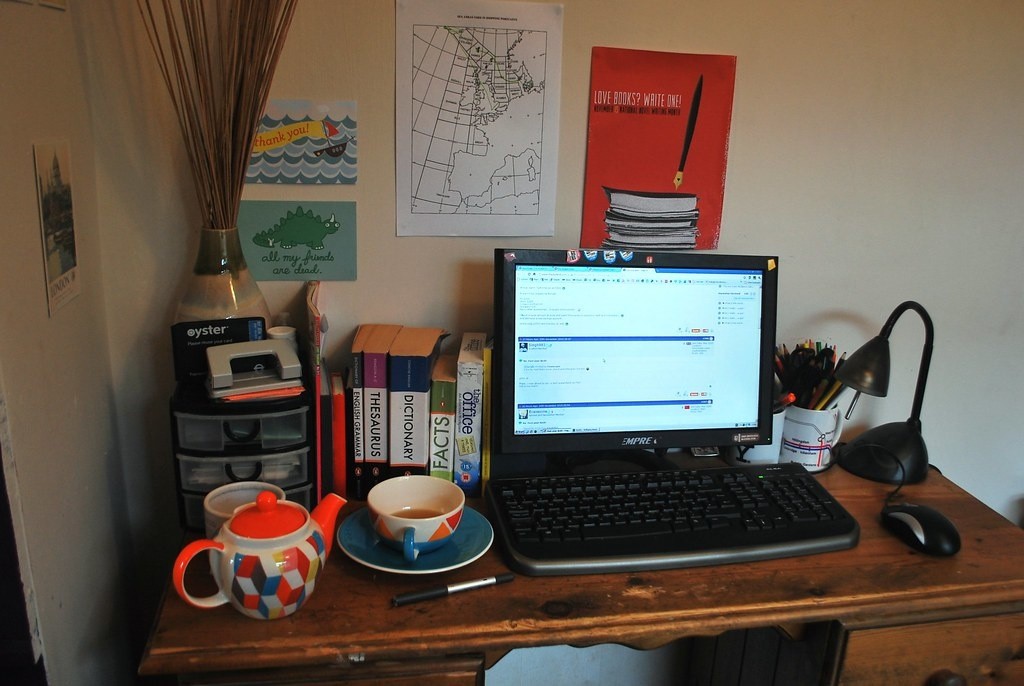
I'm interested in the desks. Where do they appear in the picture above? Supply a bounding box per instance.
[138,464,1024,686]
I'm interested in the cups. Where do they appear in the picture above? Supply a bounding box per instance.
[267,327,296,355]
[204,481,287,539]
[367,475,465,562]
[780,402,844,474]
[725,406,786,465]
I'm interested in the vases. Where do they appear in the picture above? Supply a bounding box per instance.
[174,228,271,331]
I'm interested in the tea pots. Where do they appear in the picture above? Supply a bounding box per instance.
[172,489,348,619]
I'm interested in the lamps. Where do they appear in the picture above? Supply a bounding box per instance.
[834,301,934,484]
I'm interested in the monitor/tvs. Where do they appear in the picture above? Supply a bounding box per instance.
[492,247,778,479]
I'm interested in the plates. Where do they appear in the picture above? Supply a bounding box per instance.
[337,506,493,574]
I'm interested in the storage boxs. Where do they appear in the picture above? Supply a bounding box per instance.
[453,333,487,493]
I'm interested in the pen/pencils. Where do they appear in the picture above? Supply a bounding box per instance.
[769,338,850,411]
[390,573,516,607]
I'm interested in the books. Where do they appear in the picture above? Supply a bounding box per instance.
[304,279,492,503]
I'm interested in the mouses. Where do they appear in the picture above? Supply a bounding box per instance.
[880,505,961,557]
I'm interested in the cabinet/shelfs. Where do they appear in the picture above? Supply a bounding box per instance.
[170,381,315,534]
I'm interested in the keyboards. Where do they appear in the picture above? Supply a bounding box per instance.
[486,461,861,576]
